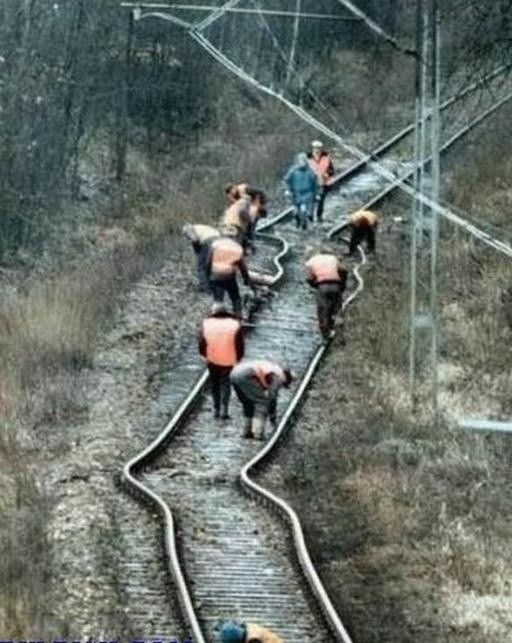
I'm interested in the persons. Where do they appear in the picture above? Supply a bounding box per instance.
[182,221,219,289]
[219,181,267,255]
[305,249,348,339]
[282,152,323,231]
[199,303,244,420]
[328,209,378,256]
[220,621,284,643]
[307,140,334,221]
[201,231,256,318]
[231,359,293,440]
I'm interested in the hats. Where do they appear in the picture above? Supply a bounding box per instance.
[284,371,292,389]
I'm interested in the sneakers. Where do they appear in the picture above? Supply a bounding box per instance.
[214,410,230,419]
[239,430,264,440]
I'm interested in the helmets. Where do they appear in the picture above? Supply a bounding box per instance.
[311,141,322,148]
[220,620,245,642]
